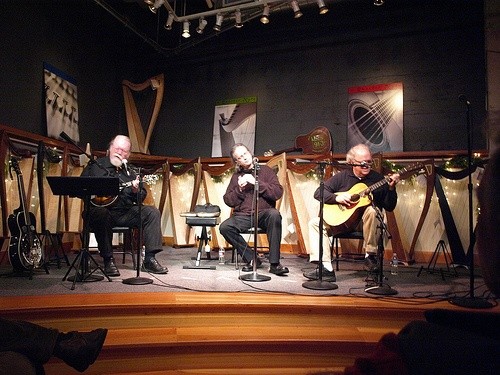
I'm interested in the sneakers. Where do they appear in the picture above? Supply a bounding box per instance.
[56,328,108,372]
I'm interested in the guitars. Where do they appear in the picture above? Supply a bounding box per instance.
[347,91,403,154]
[323,161,424,232]
[264,126,332,156]
[7,155,42,271]
[90,174,159,207]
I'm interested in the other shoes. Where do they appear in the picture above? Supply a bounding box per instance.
[364,255,378,270]
[304,266,336,281]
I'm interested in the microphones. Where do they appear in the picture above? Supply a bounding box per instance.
[458,93,470,105]
[253,156,259,165]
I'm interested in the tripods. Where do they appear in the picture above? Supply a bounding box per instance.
[46,176,119,290]
[40,148,71,268]
[428,239,460,277]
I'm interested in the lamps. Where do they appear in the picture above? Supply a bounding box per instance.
[144,0,328,39]
[373,0,383,6]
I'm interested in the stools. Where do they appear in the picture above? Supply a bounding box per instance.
[183,204,221,270]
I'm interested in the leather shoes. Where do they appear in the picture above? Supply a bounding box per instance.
[242,257,261,271]
[104,257,120,275]
[141,257,169,273]
[269,263,289,274]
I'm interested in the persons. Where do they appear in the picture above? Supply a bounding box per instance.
[0,318,108,375]
[344,141,500,375]
[303,144,400,288]
[219,143,289,274]
[79,135,168,275]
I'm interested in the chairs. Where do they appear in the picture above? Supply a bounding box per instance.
[79,226,137,275]
[232,229,269,270]
[330,230,369,272]
